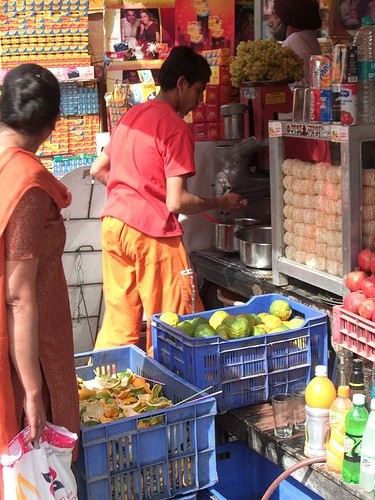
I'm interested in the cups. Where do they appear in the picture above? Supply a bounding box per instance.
[272,393,292,438]
[292,387,306,430]
[362,371,372,411]
[335,363,352,397]
[337,349,354,363]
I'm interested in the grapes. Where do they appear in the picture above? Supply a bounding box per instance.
[230,39,304,87]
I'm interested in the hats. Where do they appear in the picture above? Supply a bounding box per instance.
[274,0,323,31]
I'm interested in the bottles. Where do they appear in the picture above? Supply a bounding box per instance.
[349,360,364,400]
[303,366,337,459]
[353,15,375,125]
[341,393,369,484]
[326,385,353,473]
[358,398,375,491]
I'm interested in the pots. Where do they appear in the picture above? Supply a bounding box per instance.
[214,217,261,254]
[233,224,273,270]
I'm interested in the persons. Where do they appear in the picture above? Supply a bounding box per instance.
[90,47,249,369]
[121,8,161,44]
[1,62,80,500]
[236,7,254,42]
[268,0,322,87]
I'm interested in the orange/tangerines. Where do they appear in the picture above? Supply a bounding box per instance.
[159,300,306,389]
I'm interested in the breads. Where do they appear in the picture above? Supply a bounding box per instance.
[281,158,375,274]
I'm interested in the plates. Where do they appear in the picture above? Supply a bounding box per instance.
[239,79,297,87]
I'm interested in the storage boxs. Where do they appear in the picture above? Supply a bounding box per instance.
[73,345,216,500]
[183,47,240,142]
[198,442,321,500]
[331,305,375,362]
[151,292,329,415]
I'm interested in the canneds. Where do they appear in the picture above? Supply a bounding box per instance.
[310,43,360,125]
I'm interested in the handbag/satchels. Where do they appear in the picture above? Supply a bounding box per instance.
[1,420,79,500]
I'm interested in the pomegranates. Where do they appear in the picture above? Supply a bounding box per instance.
[343,248,375,323]
